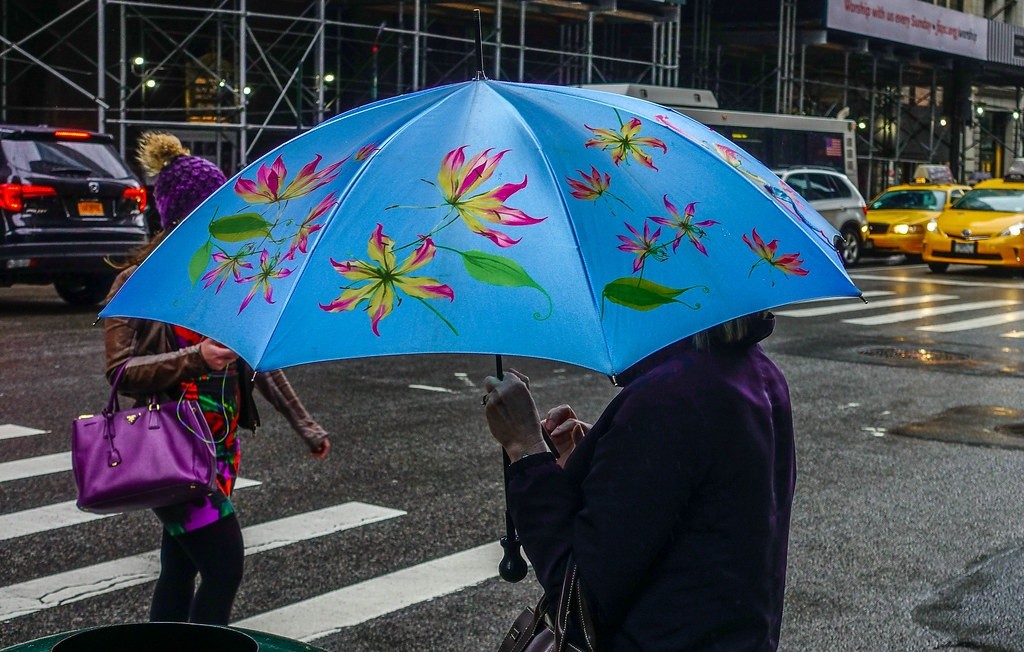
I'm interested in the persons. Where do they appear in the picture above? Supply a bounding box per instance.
[482,302,798,652]
[105,129,330,628]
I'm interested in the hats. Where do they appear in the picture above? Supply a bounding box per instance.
[133,130,227,228]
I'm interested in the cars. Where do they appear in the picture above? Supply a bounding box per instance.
[921,157,1024,274]
[855,164,974,265]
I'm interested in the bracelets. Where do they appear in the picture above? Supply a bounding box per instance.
[512,441,548,462]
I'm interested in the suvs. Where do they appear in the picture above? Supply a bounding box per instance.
[772,167,869,267]
[0,124,152,307]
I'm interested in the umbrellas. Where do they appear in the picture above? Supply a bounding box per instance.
[98,4,865,580]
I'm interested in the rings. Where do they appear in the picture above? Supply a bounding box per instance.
[481,394,489,407]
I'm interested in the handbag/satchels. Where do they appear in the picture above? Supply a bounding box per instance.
[497,558,597,652]
[71,356,217,515]
